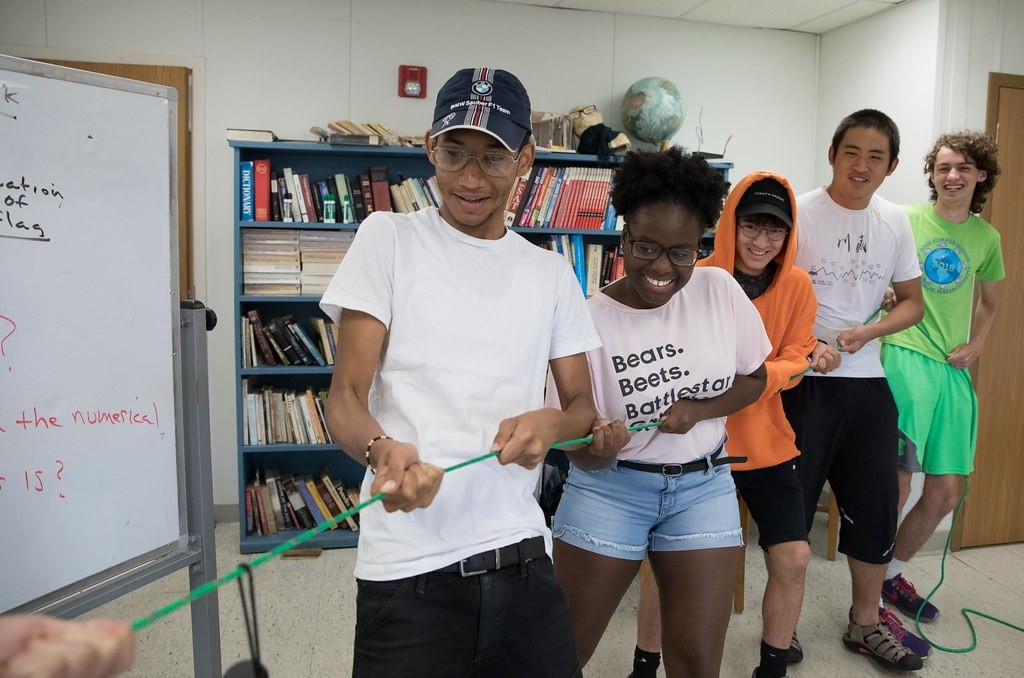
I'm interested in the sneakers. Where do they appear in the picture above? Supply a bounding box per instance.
[882,573,940,623]
[848,604,933,659]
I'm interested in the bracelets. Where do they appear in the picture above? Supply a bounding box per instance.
[366,435,398,475]
[808,339,828,360]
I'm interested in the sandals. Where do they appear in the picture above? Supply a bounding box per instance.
[843,617,923,672]
[788,629,804,662]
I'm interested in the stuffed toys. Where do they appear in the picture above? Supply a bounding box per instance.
[566,103,631,155]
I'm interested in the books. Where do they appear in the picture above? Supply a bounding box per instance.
[242,378,338,445]
[240,159,443,221]
[242,310,339,370]
[227,128,278,142]
[242,228,354,296]
[531,110,577,154]
[538,234,627,298]
[504,166,624,231]
[309,119,425,148]
[246,467,362,537]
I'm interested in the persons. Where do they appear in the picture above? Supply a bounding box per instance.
[785,108,924,672]
[628,171,818,678]
[551,146,773,678]
[849,127,1005,659]
[0,612,136,678]
[319,67,602,678]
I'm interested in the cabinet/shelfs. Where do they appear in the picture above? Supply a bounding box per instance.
[229,140,731,555]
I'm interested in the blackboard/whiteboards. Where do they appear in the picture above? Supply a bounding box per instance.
[0,52,192,615]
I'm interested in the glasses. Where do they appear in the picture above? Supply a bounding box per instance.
[432,137,525,176]
[625,226,703,267]
[570,105,597,119]
[737,220,790,240]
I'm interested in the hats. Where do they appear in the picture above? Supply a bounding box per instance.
[735,178,793,228]
[432,67,532,152]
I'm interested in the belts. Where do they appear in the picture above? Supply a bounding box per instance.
[619,436,748,476]
[431,537,546,578]
